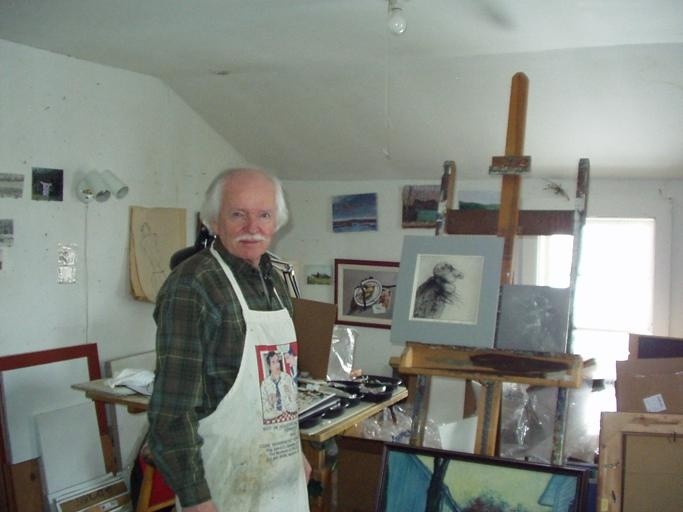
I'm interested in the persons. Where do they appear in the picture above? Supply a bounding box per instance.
[140,169,312,511]
[254,350,297,420]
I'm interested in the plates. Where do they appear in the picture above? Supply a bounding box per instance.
[354,280,382,307]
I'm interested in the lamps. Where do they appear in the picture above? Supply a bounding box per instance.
[74,165,129,205]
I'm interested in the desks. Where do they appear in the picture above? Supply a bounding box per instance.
[69,377,408,512]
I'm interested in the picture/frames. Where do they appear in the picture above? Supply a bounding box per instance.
[370,440,591,512]
[333,258,400,329]
